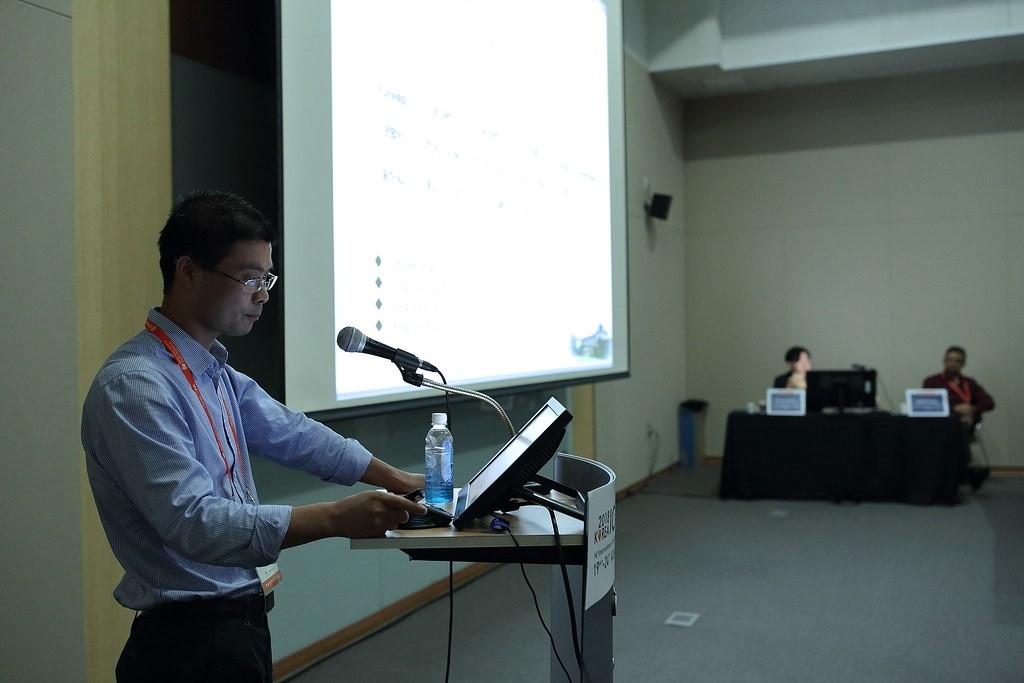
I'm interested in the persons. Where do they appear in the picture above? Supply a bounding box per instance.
[80,187,427,683]
[923,346,995,424]
[774,347,811,389]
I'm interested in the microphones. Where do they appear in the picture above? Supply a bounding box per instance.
[336,325,438,373]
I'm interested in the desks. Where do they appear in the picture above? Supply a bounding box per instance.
[720,407,970,506]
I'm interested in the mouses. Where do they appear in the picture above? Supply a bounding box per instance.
[396,502,453,530]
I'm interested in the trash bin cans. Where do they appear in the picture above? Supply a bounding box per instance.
[680,400,710,468]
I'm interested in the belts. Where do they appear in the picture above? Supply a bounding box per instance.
[141,590,275,620]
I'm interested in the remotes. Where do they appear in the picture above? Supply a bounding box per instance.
[403,488,426,503]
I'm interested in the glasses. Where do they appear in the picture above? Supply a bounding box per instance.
[179,254,278,294]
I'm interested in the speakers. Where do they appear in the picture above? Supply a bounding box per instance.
[649,193,672,220]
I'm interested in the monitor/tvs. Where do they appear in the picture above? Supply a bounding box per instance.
[805,369,877,415]
[453,397,574,532]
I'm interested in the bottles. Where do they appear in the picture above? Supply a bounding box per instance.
[424,412,455,505]
